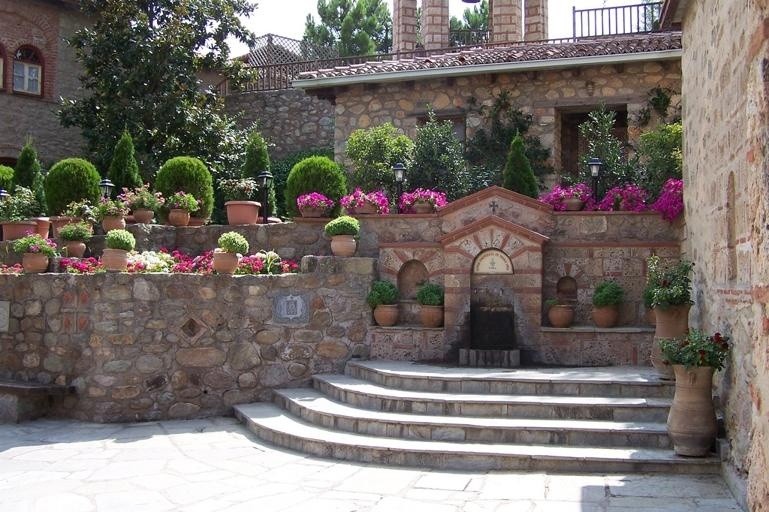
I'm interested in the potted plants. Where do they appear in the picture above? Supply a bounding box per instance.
[213,229,249,274]
[415,283,444,327]
[214,175,264,225]
[369,280,402,327]
[325,216,360,257]
[644,298,658,326]
[647,256,698,378]
[100,227,137,273]
[543,297,576,327]
[591,279,626,328]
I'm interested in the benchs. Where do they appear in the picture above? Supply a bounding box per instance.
[1,379,77,425]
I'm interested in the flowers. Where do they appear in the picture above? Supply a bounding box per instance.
[537,176,686,221]
[295,189,447,218]
[12,228,58,258]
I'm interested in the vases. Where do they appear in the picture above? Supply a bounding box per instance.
[2,184,204,257]
[654,328,730,373]
[22,252,49,274]
[667,363,720,458]
